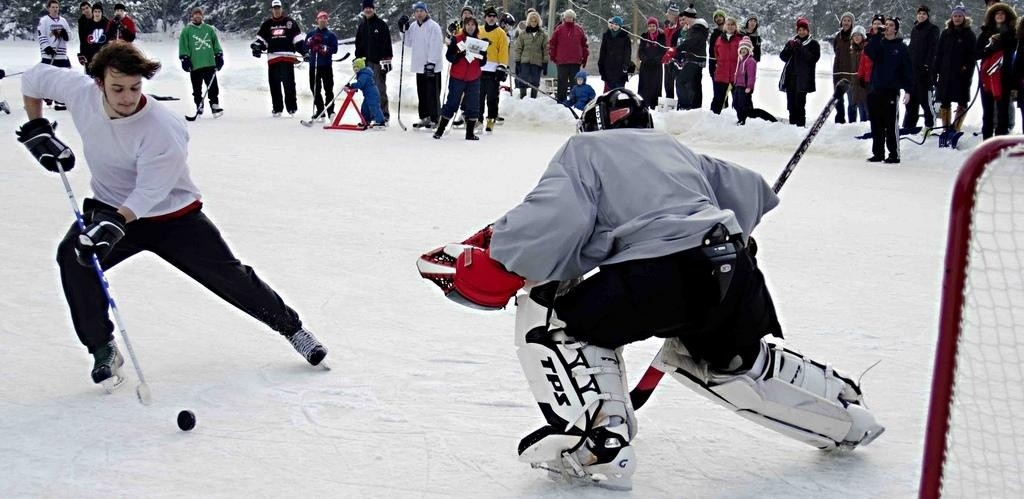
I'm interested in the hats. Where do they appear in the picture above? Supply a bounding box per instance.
[414,2,427,12]
[646,17,659,48]
[668,1,679,16]
[713,9,725,21]
[951,5,966,18]
[363,0,374,9]
[485,7,497,16]
[461,6,473,16]
[872,14,885,25]
[796,17,810,33]
[885,15,900,31]
[744,12,759,34]
[272,0,282,8]
[850,25,867,49]
[317,11,328,18]
[114,3,126,11]
[92,1,103,13]
[192,7,202,15]
[563,8,576,19]
[839,12,855,39]
[683,3,697,18]
[609,16,623,26]
[917,5,930,17]
[352,56,366,68]
[734,35,754,75]
[526,12,542,27]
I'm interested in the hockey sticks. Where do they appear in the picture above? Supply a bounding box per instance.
[398,24,409,131]
[443,93,464,135]
[261,51,351,62]
[628,78,852,412]
[506,69,580,121]
[300,73,356,128]
[184,68,217,122]
[309,52,318,125]
[54,157,155,407]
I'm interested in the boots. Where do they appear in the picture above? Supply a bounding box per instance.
[433,117,448,139]
[466,120,479,139]
[939,105,949,131]
[954,106,966,131]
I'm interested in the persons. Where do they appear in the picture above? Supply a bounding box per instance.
[432,16,488,141]
[902,5,940,132]
[36,1,72,110]
[732,35,757,125]
[603,18,614,93]
[859,14,885,122]
[663,3,680,99]
[494,13,516,126]
[666,4,710,109]
[249,0,311,118]
[398,1,444,133]
[637,16,666,110]
[710,17,743,115]
[106,3,137,44]
[777,14,821,126]
[345,57,387,131]
[562,71,596,111]
[976,3,1017,141]
[935,5,977,136]
[708,9,728,108]
[739,12,761,63]
[598,16,632,91]
[515,8,536,37]
[304,11,338,120]
[546,8,589,104]
[354,0,393,126]
[984,0,1016,134]
[415,86,885,492]
[445,5,474,130]
[832,12,857,124]
[0,70,11,115]
[473,7,509,136]
[178,7,226,119]
[512,12,549,99]
[76,2,92,43]
[850,25,874,88]
[864,17,913,164]
[16,40,332,394]
[671,10,687,110]
[77,1,111,80]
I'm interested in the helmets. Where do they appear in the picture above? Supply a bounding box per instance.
[499,12,516,24]
[575,87,653,132]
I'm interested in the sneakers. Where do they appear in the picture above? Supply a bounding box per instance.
[0,102,10,114]
[328,112,335,118]
[885,155,900,163]
[452,119,464,125]
[818,398,864,451]
[211,103,223,113]
[91,338,124,383]
[474,121,484,130]
[312,110,325,119]
[373,121,384,126]
[867,155,884,162]
[496,115,504,121]
[285,324,327,367]
[546,422,637,478]
[425,121,436,128]
[412,117,426,129]
[197,103,204,114]
[486,117,494,131]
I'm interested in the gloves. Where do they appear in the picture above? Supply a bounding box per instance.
[302,49,310,62]
[794,38,803,48]
[378,57,392,72]
[542,63,548,76]
[785,40,794,47]
[516,61,521,74]
[306,33,322,46]
[181,55,192,72]
[73,213,126,267]
[62,28,68,41]
[15,117,75,173]
[582,57,587,68]
[312,43,330,55]
[215,52,224,71]
[252,45,262,57]
[494,65,507,82]
[44,46,57,58]
[398,15,410,33]
[116,22,124,31]
[113,14,121,22]
[77,54,87,65]
[660,47,679,64]
[424,63,435,79]
